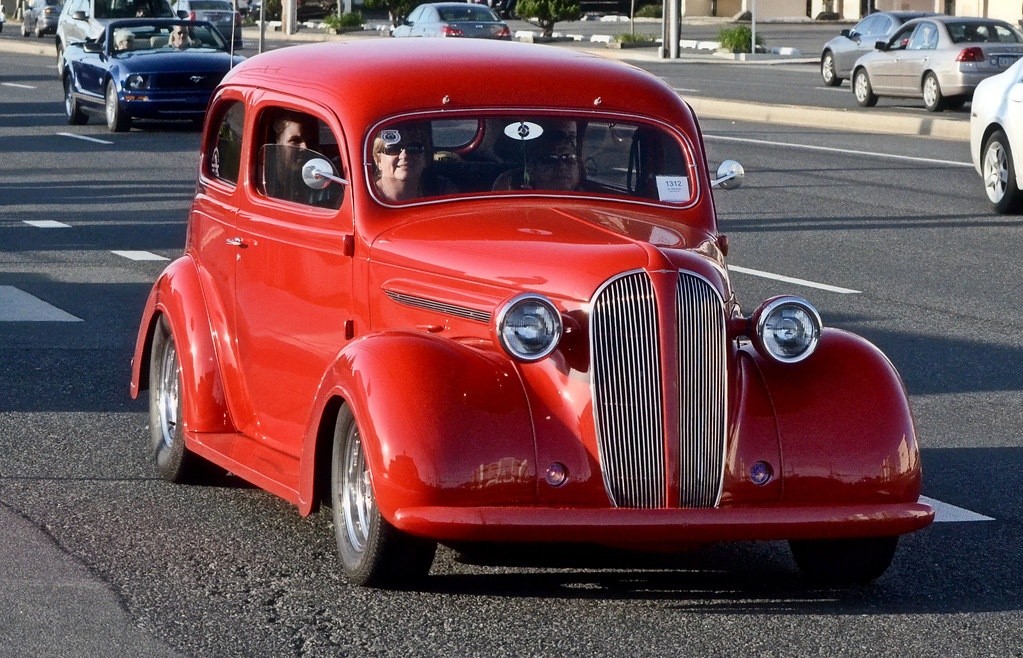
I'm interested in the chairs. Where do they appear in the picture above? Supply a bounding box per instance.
[493,167,525,190]
[150,35,168,48]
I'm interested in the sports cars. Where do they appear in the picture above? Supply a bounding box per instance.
[61,17,247,133]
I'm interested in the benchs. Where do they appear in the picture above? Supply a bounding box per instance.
[440,156,522,193]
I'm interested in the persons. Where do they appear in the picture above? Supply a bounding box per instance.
[269,111,333,205]
[163,25,203,48]
[372,125,459,202]
[115,28,135,50]
[534,132,580,192]
[492,120,576,192]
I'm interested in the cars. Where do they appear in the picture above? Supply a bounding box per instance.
[821,10,986,87]
[21,0,66,38]
[850,16,1023,112]
[392,2,512,42]
[970,57,1023,215]
[171,0,243,50]
[130,38,935,589]
[56,0,189,80]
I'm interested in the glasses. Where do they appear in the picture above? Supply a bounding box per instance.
[122,39,133,43]
[539,153,578,165]
[381,141,426,156]
[177,31,189,35]
